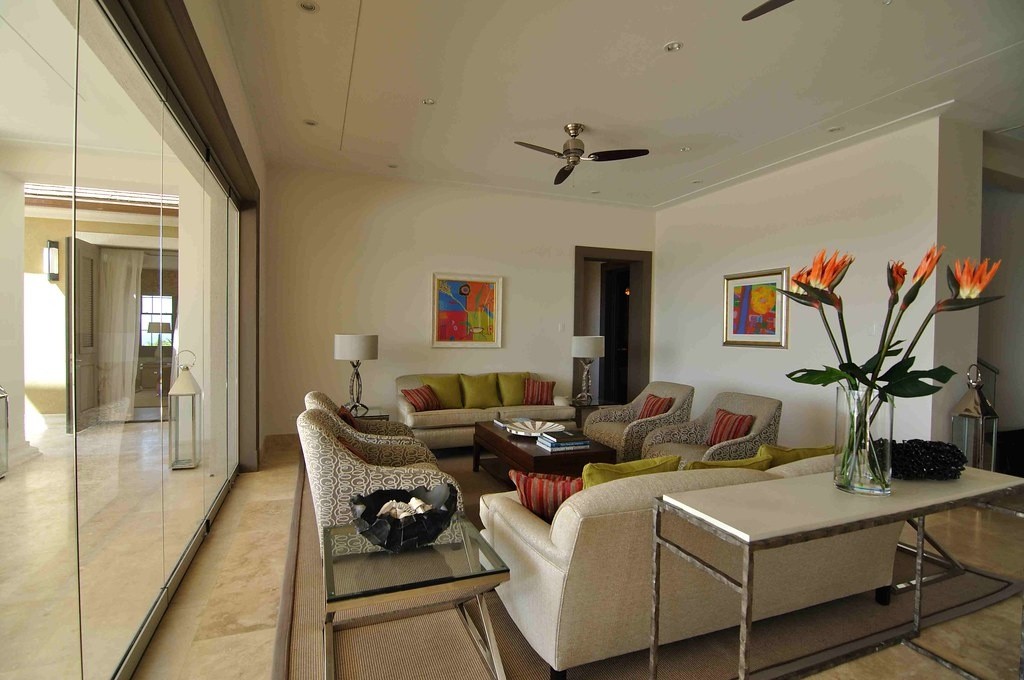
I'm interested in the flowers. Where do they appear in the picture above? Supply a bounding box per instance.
[772,243,1003,493]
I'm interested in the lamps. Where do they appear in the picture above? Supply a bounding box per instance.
[147,323,170,399]
[570,336,605,402]
[333,334,378,412]
[47,240,59,280]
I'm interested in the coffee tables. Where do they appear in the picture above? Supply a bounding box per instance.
[472,420,617,490]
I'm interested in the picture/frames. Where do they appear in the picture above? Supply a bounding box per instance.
[431,271,503,349]
[723,266,790,350]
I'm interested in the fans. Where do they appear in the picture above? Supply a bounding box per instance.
[741,0,796,21]
[514,122,650,186]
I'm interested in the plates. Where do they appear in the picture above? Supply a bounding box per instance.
[506,421,565,436]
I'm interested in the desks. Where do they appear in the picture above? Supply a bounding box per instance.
[647,462,1024,680]
[568,400,612,429]
[322,510,510,680]
[348,409,389,423]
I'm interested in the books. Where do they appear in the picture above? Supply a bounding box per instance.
[494,417,533,427]
[537,431,592,452]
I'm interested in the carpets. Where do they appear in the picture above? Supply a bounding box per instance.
[271,446,1024,680]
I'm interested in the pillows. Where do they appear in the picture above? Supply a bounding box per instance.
[706,409,753,443]
[688,456,772,472]
[337,405,364,434]
[401,371,556,412]
[757,446,841,468]
[583,456,683,490]
[639,395,673,421]
[509,470,583,523]
[337,436,369,464]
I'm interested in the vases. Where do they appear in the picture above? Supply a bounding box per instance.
[834,386,893,497]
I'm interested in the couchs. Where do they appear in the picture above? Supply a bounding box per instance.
[584,380,694,463]
[479,449,906,680]
[641,391,783,471]
[396,371,843,523]
[304,390,427,445]
[297,408,465,560]
